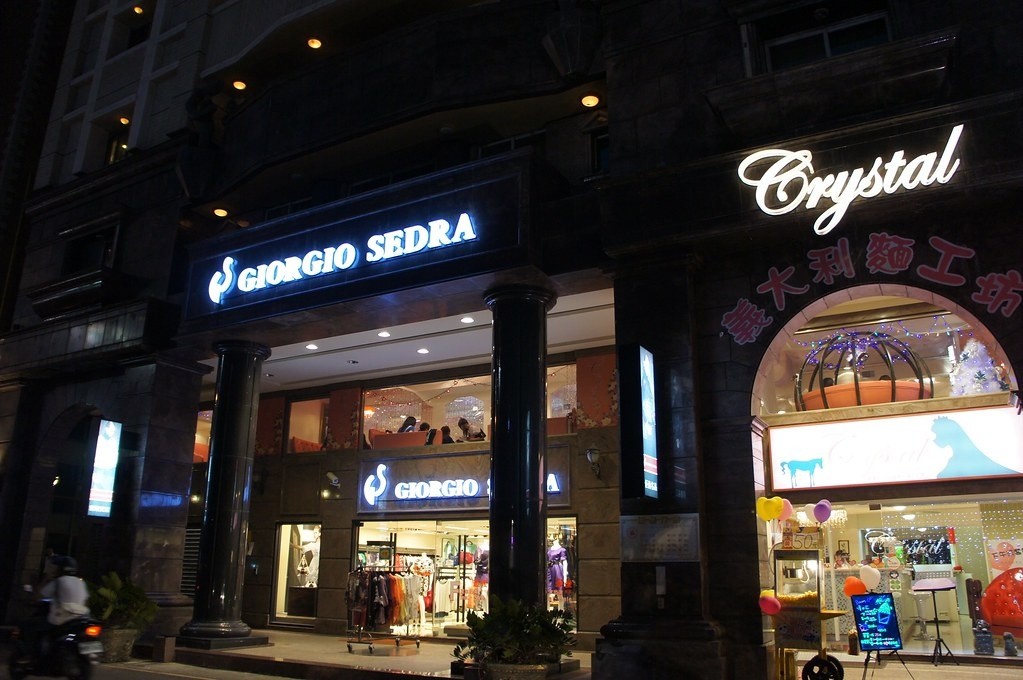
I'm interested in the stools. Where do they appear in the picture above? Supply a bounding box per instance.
[908,589,937,643]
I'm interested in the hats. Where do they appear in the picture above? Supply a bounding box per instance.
[58,555,80,569]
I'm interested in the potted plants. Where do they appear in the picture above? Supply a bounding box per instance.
[451,594,579,680]
[86,572,163,664]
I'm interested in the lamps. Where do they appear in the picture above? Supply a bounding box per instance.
[782,566,804,579]
[587,448,602,480]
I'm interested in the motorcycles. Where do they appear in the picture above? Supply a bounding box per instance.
[7,585,105,680]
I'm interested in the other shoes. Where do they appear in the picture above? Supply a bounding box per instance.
[16,652,36,664]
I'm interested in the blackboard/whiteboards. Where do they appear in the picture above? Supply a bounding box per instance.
[850,593,904,652]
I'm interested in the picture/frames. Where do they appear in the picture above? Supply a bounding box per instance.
[838,540,850,555]
[317,402,329,445]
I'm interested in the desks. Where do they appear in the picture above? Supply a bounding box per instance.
[911,585,961,667]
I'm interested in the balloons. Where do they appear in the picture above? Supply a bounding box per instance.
[804,500,832,524]
[760,590,781,615]
[757,495,792,523]
[843,566,881,597]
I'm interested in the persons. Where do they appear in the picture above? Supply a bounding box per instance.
[417,553,435,592]
[824,378,834,387]
[291,525,320,586]
[30,557,91,676]
[386,417,486,445]
[880,375,891,380]
[548,539,567,601]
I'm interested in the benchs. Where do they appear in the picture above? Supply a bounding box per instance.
[291,436,323,454]
[373,431,429,448]
[799,380,933,409]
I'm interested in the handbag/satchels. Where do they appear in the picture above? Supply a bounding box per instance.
[446,541,477,566]
